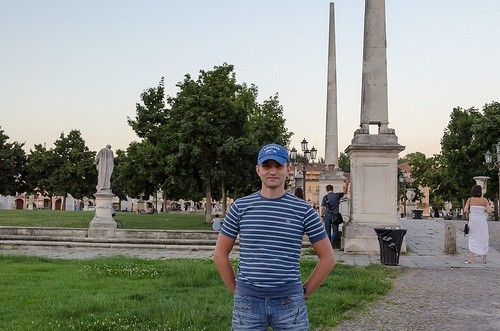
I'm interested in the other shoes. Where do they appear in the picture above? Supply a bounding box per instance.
[331,241,335,245]
[480,260,486,264]
[464,259,476,264]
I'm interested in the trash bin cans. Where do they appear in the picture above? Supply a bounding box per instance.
[374,228,407,266]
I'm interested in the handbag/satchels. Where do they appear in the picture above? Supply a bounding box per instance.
[464,224,469,235]
[331,213,343,224]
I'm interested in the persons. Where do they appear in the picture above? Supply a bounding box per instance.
[211,214,223,231]
[94,144,114,189]
[464,185,491,264]
[321,176,350,247]
[212,143,336,331]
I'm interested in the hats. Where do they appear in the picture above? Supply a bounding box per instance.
[257,144,290,166]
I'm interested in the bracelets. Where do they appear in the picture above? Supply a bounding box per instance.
[302,285,308,297]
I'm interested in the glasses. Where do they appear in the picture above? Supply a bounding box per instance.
[259,164,287,171]
